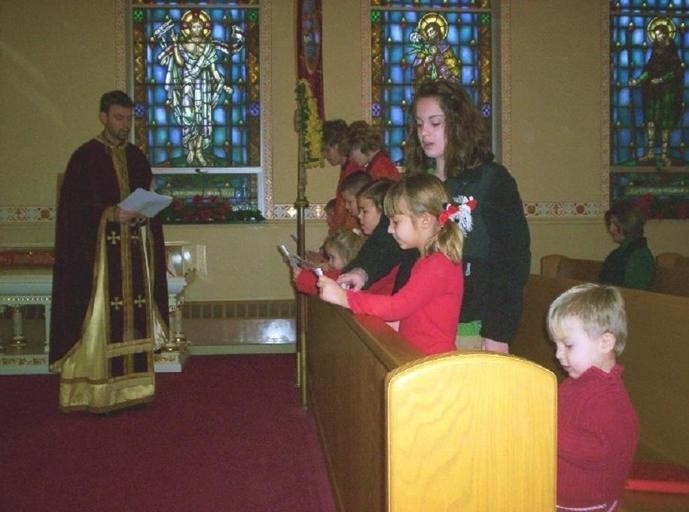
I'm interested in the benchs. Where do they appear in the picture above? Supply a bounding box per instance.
[509,252,689,512]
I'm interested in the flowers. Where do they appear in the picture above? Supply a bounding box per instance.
[172,194,233,225]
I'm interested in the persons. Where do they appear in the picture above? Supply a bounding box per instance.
[596,203,655,290]
[170,18,237,166]
[290,119,402,297]
[628,24,684,166]
[545,283,638,512]
[335,81,530,352]
[316,174,475,355]
[49,91,168,378]
[412,23,461,97]
